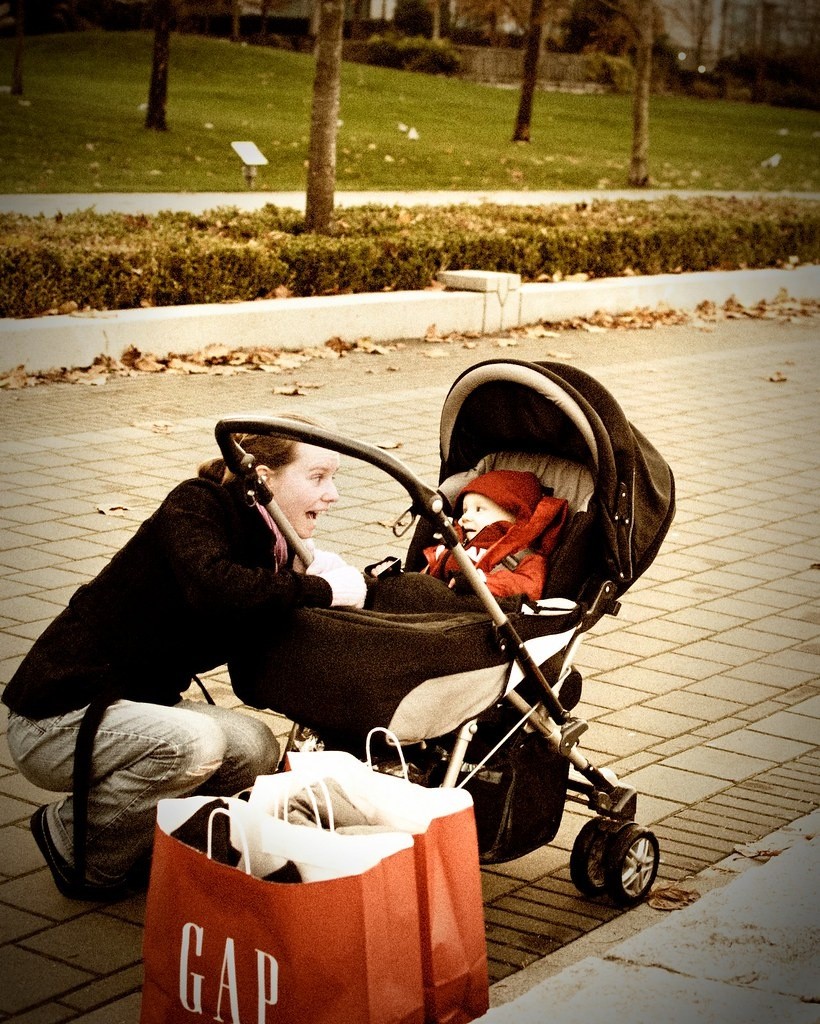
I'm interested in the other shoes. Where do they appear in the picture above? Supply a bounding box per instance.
[29,803,125,901]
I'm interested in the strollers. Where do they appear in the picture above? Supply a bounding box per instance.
[214,360,677,907]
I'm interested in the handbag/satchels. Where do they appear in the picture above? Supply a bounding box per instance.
[249,726,490,1024]
[139,767,427,1023]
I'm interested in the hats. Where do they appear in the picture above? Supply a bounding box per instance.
[455,469,543,523]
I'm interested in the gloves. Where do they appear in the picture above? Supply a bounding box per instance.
[292,537,367,609]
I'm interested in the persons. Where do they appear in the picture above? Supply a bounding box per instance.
[0,414,367,902]
[414,471,548,601]
